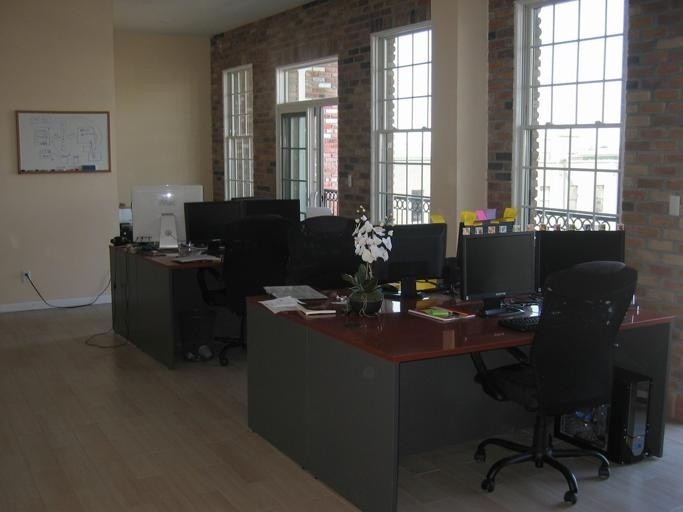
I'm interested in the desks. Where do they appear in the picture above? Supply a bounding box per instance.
[308,303,675,510]
[248,291,531,453]
[110,241,224,365]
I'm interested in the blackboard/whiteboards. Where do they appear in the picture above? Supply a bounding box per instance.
[15,110,111,175]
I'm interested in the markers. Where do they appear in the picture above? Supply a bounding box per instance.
[22,169,79,173]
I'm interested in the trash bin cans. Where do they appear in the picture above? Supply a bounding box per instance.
[179,308,216,361]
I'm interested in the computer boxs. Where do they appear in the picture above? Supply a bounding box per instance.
[553,367,654,464]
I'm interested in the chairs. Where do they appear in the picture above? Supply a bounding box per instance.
[196,214,365,367]
[468,260,640,505]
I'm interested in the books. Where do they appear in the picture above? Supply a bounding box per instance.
[297,298,337,315]
[408,304,477,321]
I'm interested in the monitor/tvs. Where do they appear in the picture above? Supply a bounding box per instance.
[184,199,300,255]
[132,185,204,253]
[372,223,448,300]
[460,232,536,318]
[536,231,624,296]
[456,218,515,268]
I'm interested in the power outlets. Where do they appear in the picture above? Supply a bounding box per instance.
[21,270,32,283]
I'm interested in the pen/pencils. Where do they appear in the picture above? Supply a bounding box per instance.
[188,240,192,252]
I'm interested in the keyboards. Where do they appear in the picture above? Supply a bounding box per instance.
[498,316,541,332]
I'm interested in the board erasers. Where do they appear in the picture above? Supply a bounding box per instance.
[81,165,95,172]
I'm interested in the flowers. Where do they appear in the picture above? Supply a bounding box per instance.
[341,205,395,294]
